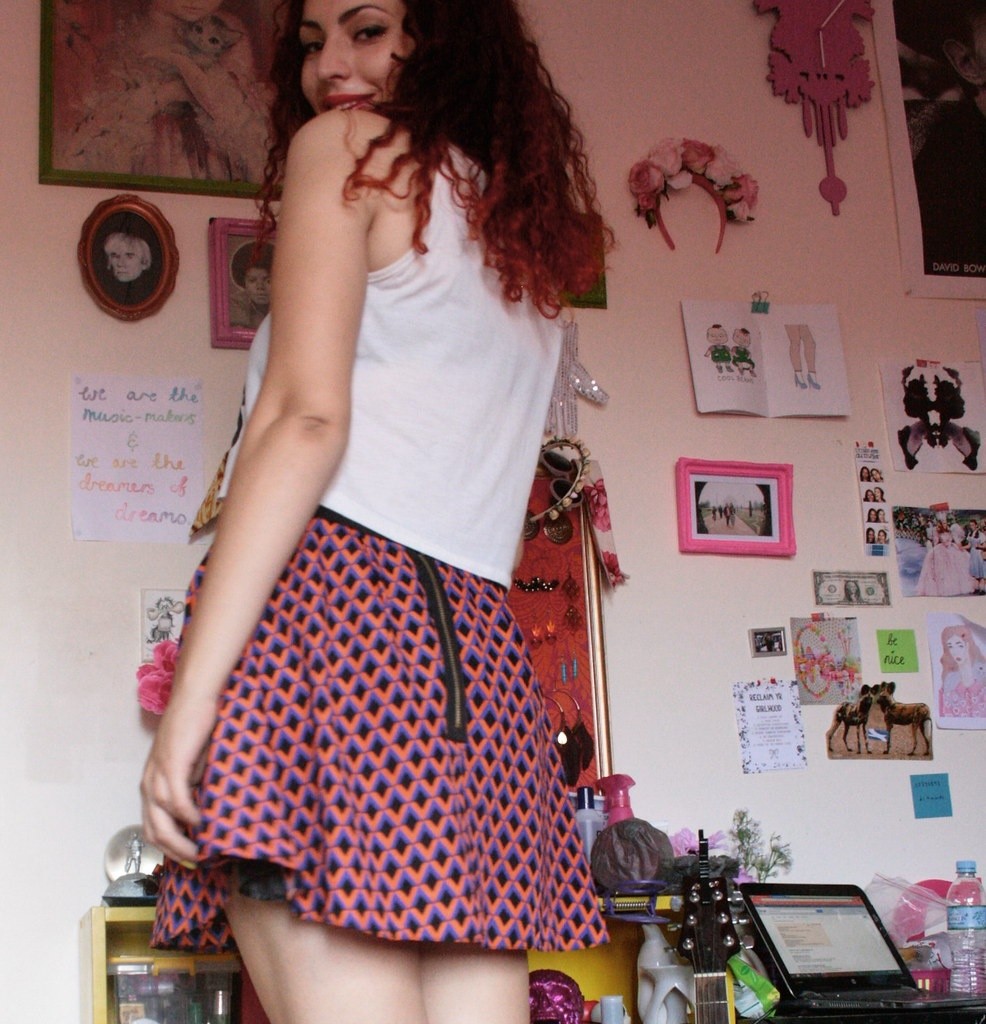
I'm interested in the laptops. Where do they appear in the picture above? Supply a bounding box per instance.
[737,883,985,1009]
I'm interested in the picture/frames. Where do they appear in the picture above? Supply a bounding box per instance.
[77,195,180,322]
[38,0,318,200]
[749,627,787,657]
[674,459,798,553]
[207,215,282,350]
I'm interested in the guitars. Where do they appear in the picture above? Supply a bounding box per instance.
[665,829,759,1024]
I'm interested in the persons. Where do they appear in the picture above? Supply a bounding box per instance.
[98,214,157,307]
[140,0,612,1024]
[230,240,277,327]
[860,466,889,544]
[711,502,736,528]
[764,633,774,651]
[917,0,986,277]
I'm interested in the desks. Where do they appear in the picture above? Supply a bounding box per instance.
[80,904,646,1024]
[774,1005,986,1024]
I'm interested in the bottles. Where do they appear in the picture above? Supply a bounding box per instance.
[945,861,986,997]
[576,786,604,864]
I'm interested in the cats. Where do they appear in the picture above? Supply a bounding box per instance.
[153,14,244,81]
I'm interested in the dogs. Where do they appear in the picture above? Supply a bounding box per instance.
[824,680,935,762]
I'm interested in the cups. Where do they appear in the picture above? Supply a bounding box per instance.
[599,995,625,1024]
